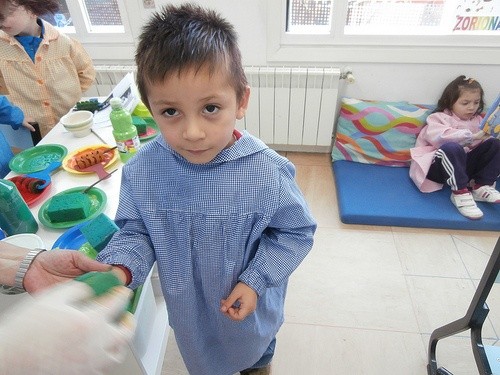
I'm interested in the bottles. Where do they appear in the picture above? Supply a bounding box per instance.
[0,179,39,236]
[110,98,141,164]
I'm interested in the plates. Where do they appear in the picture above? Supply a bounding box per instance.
[8,144,68,174]
[38,185,107,229]
[51,220,89,251]
[135,117,160,141]
[62,143,119,174]
[6,175,51,206]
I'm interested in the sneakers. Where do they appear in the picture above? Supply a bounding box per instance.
[450,191,484,220]
[472,185,500,203]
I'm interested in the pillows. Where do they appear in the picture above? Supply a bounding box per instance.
[330,97,438,168]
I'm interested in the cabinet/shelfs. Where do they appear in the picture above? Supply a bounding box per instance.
[0,95,172,375]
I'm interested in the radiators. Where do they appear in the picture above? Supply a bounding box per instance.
[83,66,354,153]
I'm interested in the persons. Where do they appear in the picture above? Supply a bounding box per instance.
[408,76,500,220]
[0,0,95,148]
[93,2,318,375]
[0,242,136,375]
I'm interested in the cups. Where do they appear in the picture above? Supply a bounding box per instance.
[0,233,43,250]
[60,110,93,138]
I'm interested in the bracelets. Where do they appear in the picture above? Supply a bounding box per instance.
[14,248,47,291]
